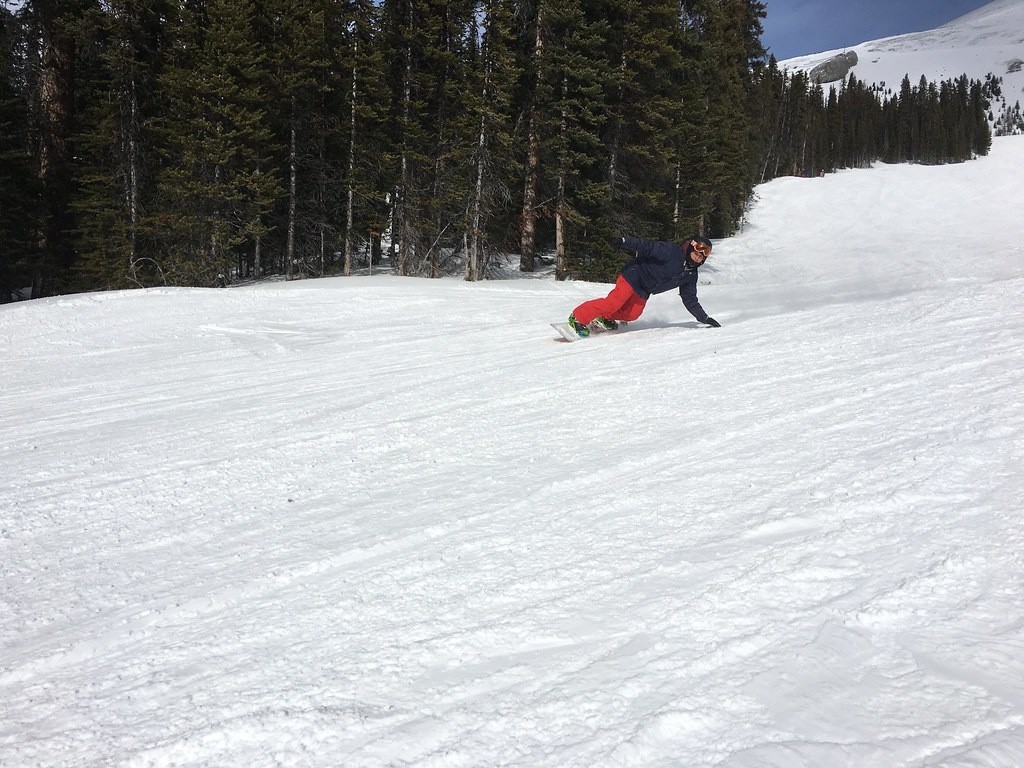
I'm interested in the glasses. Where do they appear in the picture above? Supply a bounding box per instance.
[690,239,711,256]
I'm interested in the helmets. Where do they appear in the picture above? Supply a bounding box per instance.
[686,235,712,269]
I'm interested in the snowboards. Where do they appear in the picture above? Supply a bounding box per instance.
[550,317,625,343]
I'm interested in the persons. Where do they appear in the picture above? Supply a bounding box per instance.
[569,235,721,336]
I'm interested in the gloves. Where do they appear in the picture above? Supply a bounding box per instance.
[705,317,721,327]
[608,236,623,253]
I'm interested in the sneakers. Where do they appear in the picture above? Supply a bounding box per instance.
[568,313,590,335]
[594,314,618,331]
